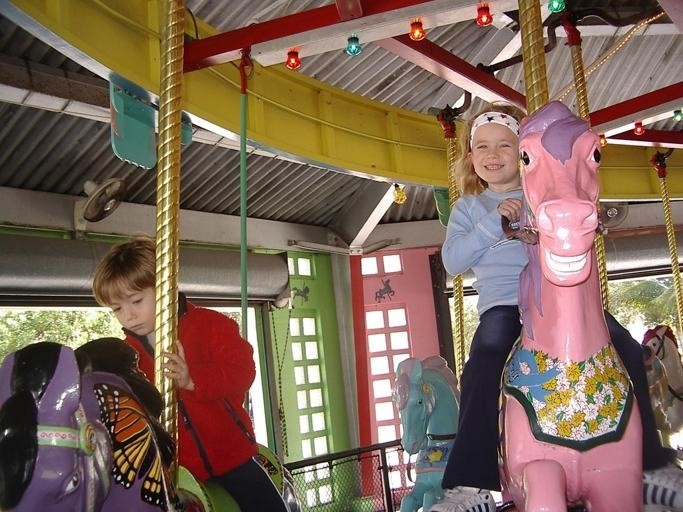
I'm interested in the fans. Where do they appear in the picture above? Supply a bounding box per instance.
[74,178,127,231]
[601,202,629,235]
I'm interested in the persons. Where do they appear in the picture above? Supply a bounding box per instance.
[428,105,683,512]
[93,237,287,512]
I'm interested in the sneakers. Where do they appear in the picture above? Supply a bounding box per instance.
[428,486,496,512]
[643,465,683,512]
